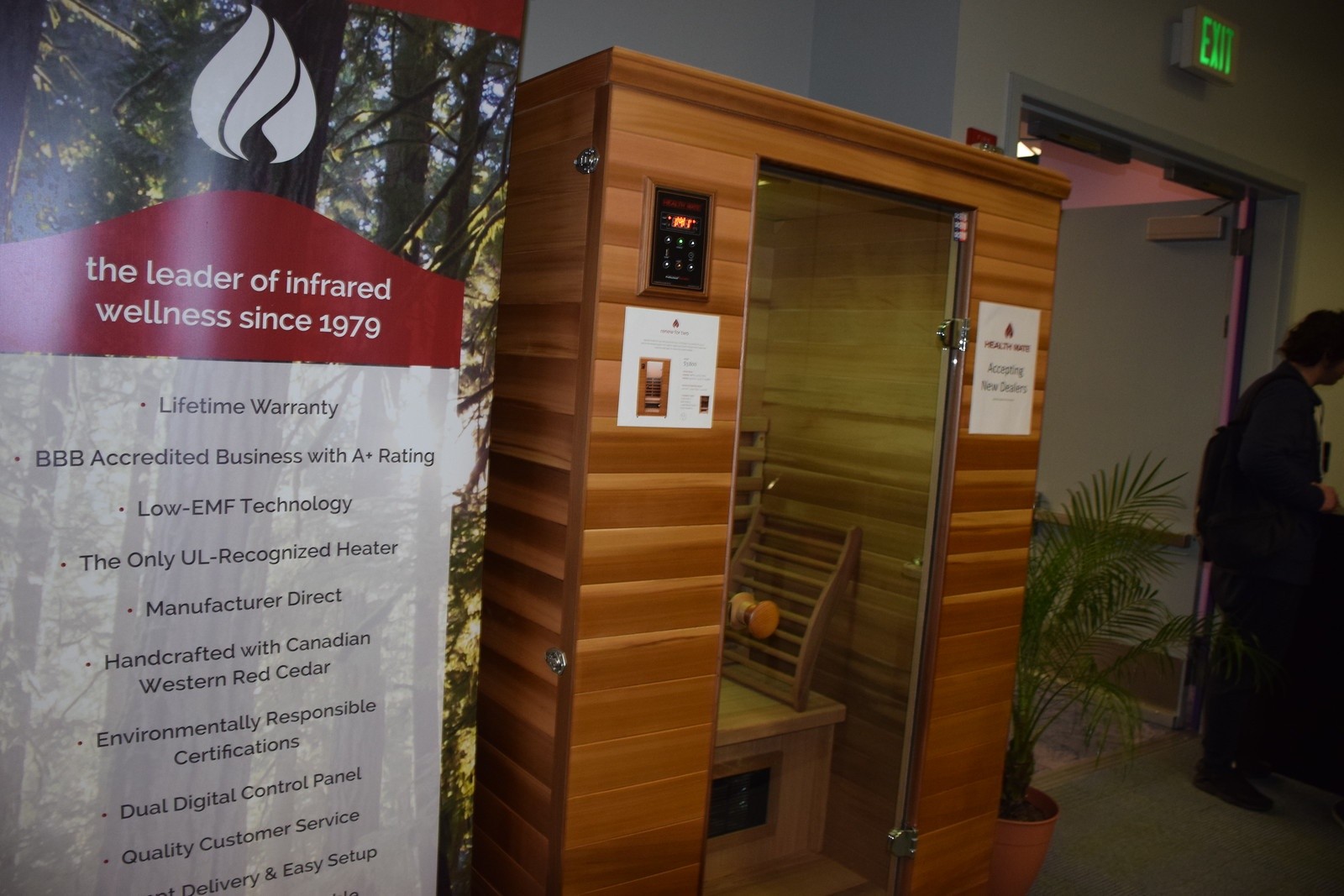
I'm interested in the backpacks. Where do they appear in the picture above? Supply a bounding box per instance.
[1193,375,1312,567]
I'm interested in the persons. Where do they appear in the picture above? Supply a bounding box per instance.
[1193,310,1344,812]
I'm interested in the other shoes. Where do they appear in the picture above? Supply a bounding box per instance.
[1330,797,1343,827]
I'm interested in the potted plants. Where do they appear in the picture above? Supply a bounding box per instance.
[992,450,1281,896]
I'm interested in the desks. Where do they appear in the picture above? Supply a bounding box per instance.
[705,676,849,889]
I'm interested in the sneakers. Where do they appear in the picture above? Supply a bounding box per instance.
[1233,749,1271,781]
[1192,762,1272,811]
